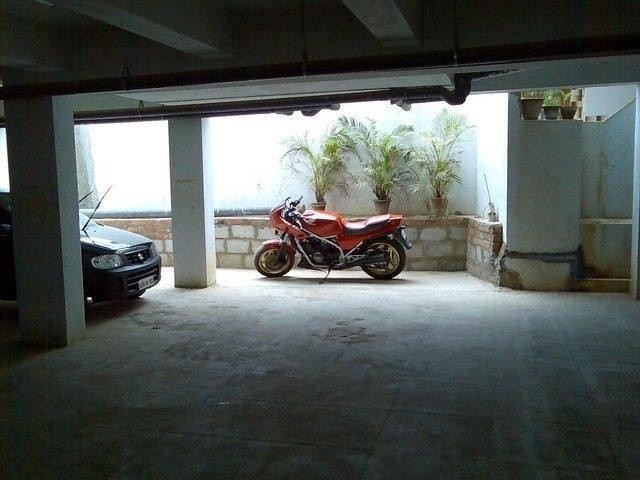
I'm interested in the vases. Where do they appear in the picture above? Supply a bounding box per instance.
[521,90,580,120]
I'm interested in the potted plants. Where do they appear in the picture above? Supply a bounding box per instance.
[278,107,477,217]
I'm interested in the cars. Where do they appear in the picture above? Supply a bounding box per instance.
[1,187,165,320]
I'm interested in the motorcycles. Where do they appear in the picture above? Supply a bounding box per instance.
[253,194,414,282]
